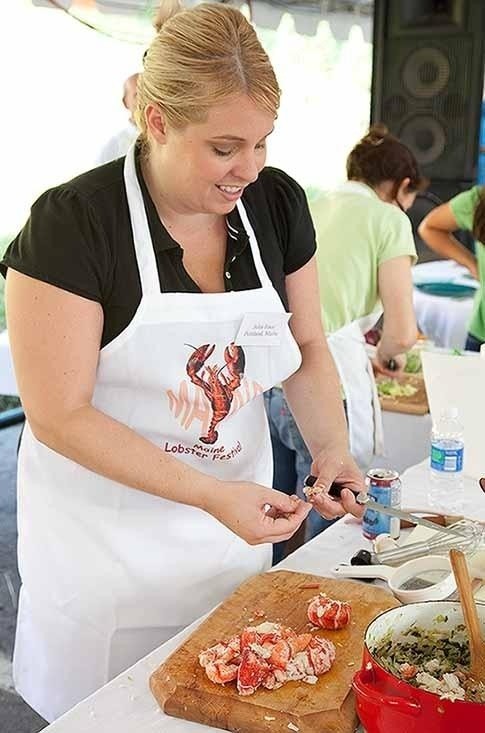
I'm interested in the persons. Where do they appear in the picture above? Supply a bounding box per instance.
[0,0,369,727]
[265,122,427,567]
[417,183,485,351]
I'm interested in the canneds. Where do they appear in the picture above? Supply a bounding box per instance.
[361,467,402,543]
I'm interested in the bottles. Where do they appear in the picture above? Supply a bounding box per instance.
[427,407,464,513]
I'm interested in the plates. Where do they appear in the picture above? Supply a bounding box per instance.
[411,281,476,297]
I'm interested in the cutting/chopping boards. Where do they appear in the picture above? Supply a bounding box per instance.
[375,370,429,415]
[148,570,404,733]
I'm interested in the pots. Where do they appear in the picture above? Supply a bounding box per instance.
[352,600,484,733]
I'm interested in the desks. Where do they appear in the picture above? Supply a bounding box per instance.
[405,259,480,350]
[364,345,482,477]
[35,441,484,733]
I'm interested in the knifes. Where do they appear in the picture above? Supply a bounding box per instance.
[303,475,465,538]
